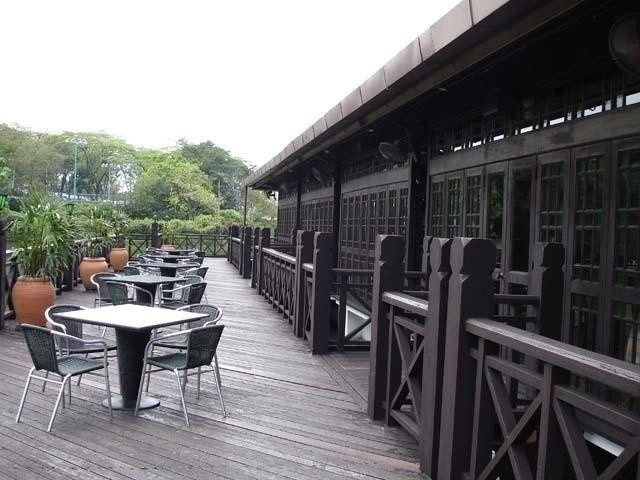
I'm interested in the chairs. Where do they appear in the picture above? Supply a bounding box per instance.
[15,323,113,431]
[90,243,209,331]
[134,325,226,426]
[145,303,223,405]
[42,304,117,404]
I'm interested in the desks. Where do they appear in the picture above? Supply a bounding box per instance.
[53,303,210,411]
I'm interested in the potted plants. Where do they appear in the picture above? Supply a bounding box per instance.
[101,209,134,270]
[157,218,184,253]
[6,202,73,329]
[69,204,111,292]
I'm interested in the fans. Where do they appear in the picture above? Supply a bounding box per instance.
[378,139,418,165]
[311,166,335,185]
